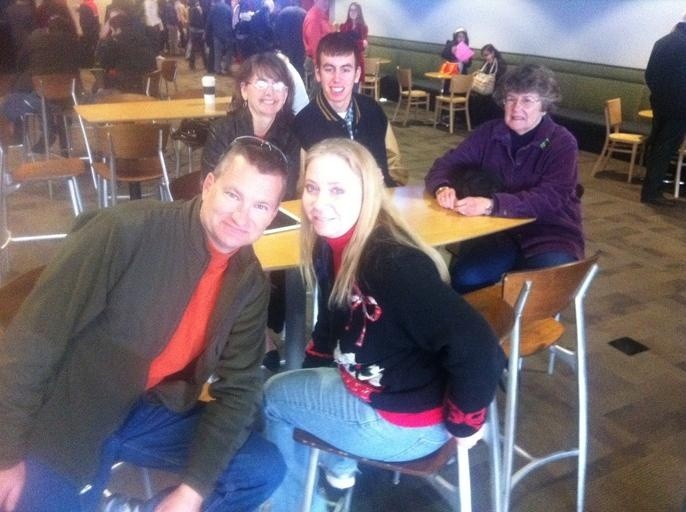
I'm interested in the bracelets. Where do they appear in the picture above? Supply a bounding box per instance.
[261,138,508,512]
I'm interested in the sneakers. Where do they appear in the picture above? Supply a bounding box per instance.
[261,342,280,372]
[104,494,142,512]
[316,450,355,490]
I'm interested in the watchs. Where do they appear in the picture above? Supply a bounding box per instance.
[485,198,493,216]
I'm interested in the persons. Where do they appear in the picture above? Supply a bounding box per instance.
[640,12,686,207]
[207,1,233,75]
[295,33,408,188]
[200,53,300,201]
[251,0,276,51]
[472,45,507,96]
[440,28,473,95]
[233,0,256,63]
[303,1,332,102]
[276,0,307,70]
[95,15,157,94]
[76,0,99,68]
[0,12,80,151]
[339,3,368,93]
[435,186,449,196]
[143,1,207,69]
[424,64,586,293]
[1,136,289,512]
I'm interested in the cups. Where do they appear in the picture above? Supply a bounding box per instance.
[202,77,215,103]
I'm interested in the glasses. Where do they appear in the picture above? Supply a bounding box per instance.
[502,95,538,107]
[220,136,288,166]
[351,9,357,12]
[246,79,286,91]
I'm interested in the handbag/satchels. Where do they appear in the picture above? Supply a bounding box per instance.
[471,57,497,95]
[441,61,462,76]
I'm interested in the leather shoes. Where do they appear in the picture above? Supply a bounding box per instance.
[640,196,675,206]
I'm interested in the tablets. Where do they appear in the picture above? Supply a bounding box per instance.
[264,207,300,238]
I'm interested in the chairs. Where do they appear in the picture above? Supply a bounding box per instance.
[172,117,211,177]
[358,61,380,102]
[673,137,686,199]
[590,98,649,183]
[92,125,172,202]
[0,116,85,249]
[293,290,514,512]
[433,75,474,133]
[392,65,431,127]
[463,249,601,511]
[146,59,180,97]
[26,75,87,197]
